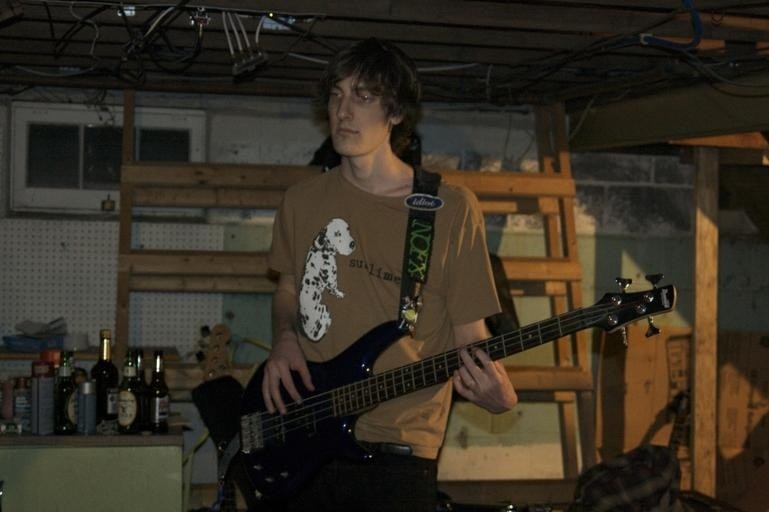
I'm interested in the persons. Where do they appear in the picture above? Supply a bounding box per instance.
[262,44,518,511]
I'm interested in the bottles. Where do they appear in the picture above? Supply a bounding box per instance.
[117,351,143,434]
[132,348,146,429]
[55,352,77,434]
[147,351,169,433]
[13,378,31,433]
[77,381,98,435]
[91,330,116,421]
[31,360,55,436]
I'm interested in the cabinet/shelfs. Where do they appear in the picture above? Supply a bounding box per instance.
[0,427,184,512]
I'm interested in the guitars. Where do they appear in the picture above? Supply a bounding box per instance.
[191,271,678,508]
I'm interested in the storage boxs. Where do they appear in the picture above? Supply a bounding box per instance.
[597,320,769,512]
